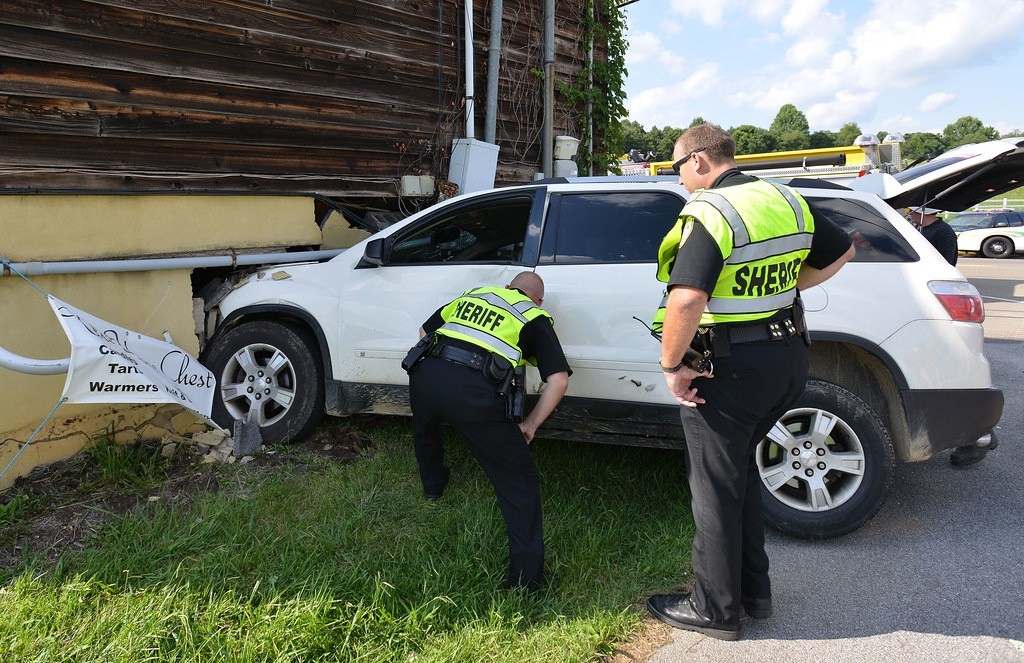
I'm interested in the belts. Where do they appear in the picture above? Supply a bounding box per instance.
[428,342,486,371]
[729,314,798,343]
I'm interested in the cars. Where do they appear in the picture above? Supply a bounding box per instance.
[950,211,1024,258]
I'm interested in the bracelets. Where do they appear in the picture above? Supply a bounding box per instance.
[658,358,682,373]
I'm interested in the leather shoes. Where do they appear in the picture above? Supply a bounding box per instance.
[422,465,451,501]
[743,591,772,620]
[950,428,999,467]
[646,593,742,641]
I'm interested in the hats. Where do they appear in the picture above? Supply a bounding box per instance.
[908,206,942,215]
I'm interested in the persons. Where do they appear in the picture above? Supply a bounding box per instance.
[911,207,958,267]
[408,271,573,592]
[646,123,856,641]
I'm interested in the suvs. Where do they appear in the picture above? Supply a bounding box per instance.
[198,136,1024,542]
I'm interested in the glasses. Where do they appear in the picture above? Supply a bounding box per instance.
[673,146,708,173]
